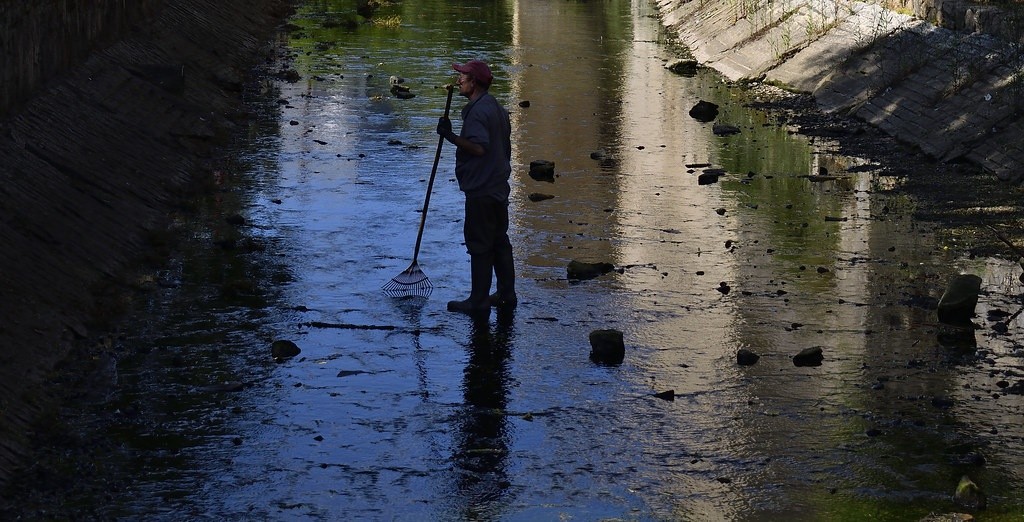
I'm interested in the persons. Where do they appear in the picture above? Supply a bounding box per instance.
[436,60,517,318]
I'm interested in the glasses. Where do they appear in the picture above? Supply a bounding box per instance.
[457,78,471,85]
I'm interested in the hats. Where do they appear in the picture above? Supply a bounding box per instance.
[453,61,492,83]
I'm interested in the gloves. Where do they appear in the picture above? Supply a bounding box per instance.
[437,117,457,144]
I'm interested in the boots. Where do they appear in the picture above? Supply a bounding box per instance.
[448,252,493,313]
[491,252,517,305]
